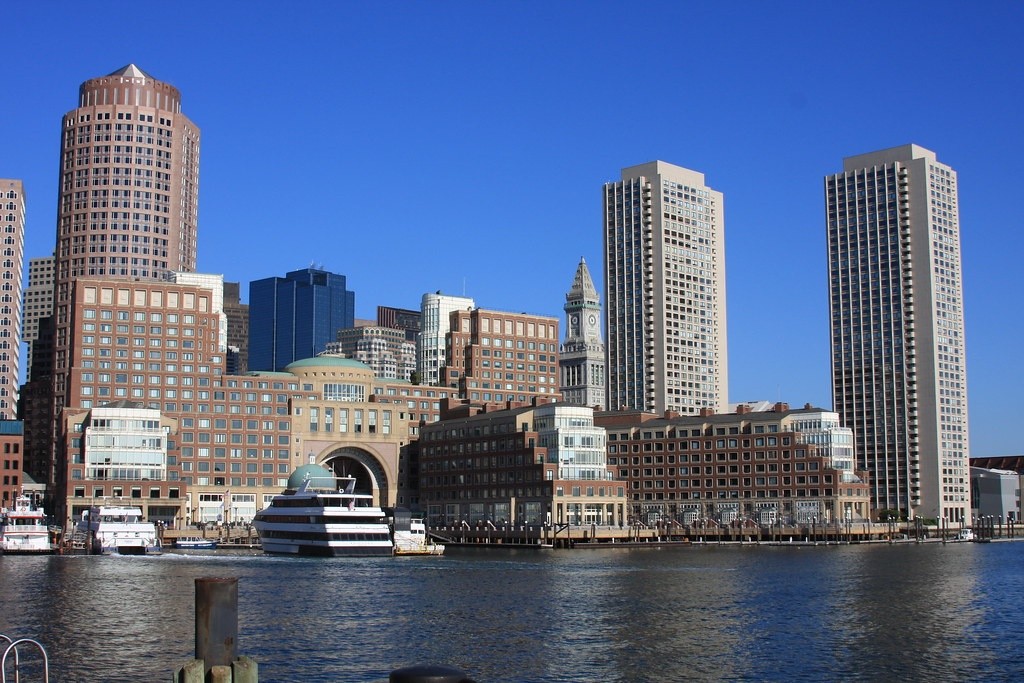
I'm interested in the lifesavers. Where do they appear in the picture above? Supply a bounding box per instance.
[21,507,26,511]
[339,489,344,493]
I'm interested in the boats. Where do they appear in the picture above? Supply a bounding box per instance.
[0,484,58,555]
[174,535,221,550]
[251,472,393,558]
[380,505,445,555]
[76,493,163,555]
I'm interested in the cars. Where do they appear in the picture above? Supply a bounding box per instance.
[954,529,977,540]
